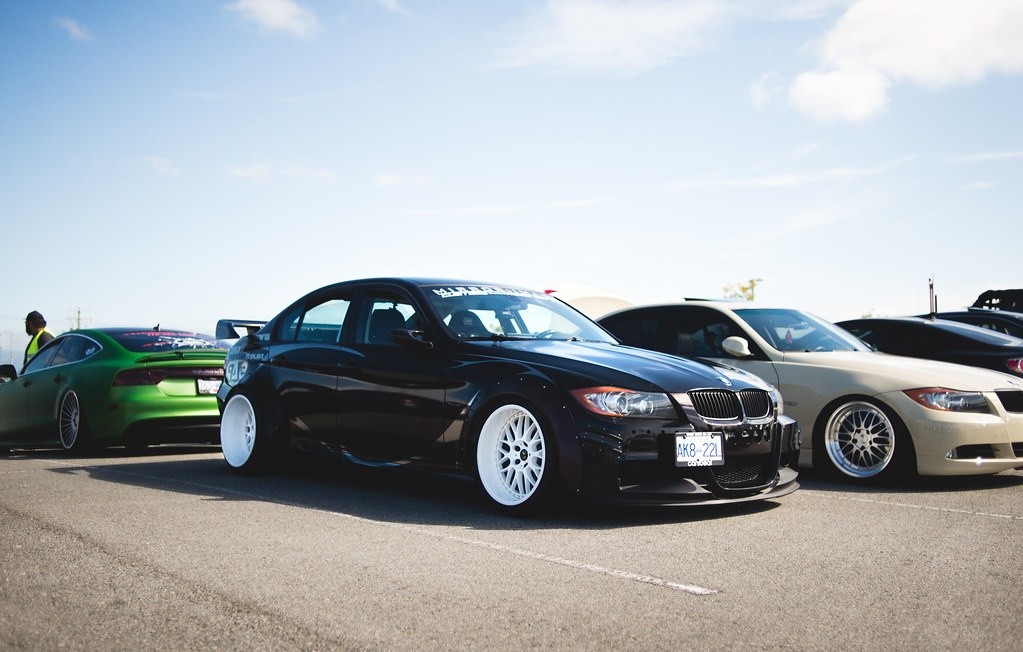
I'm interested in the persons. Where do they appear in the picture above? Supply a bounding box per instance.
[23,311,56,366]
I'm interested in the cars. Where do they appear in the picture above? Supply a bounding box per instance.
[548,296,1023,489]
[0,324,234,458]
[216,277,800,521]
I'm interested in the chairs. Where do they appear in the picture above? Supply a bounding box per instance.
[448,310,496,338]
[368,308,406,344]
